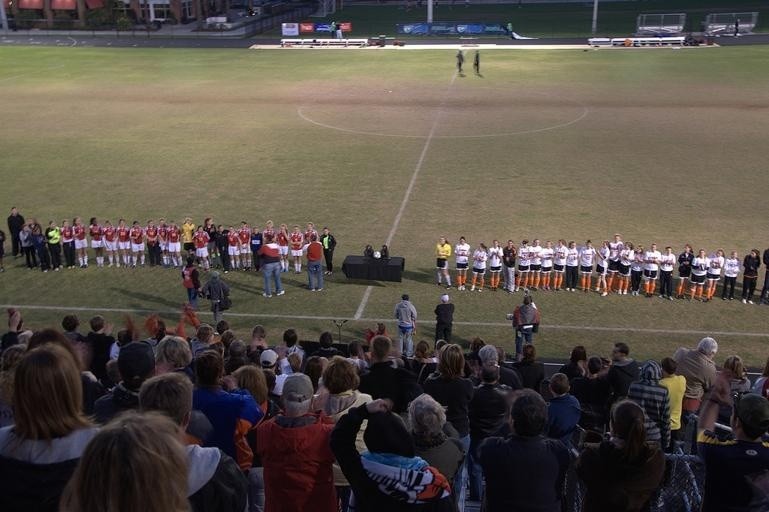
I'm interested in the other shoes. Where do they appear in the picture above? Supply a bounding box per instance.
[722,294,754,304]
[124,263,210,271]
[438,282,577,294]
[281,267,333,276]
[581,286,713,302]
[26,263,121,273]
[214,265,258,274]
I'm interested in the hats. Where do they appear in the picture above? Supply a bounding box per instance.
[282,372,314,402]
[116,340,156,380]
[229,339,247,353]
[260,348,279,367]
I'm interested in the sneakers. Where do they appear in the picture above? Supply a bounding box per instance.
[311,288,316,291]
[263,292,273,299]
[317,288,324,292]
[276,290,286,296]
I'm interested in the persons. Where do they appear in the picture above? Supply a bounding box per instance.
[1,207,336,275]
[202,271,230,326]
[473,50,480,74]
[456,50,465,72]
[330,20,337,38]
[257,235,286,298]
[513,296,541,355]
[454,236,598,294]
[394,295,417,358]
[0,307,767,512]
[182,257,202,307]
[335,21,344,39]
[595,233,768,308]
[733,19,740,38]
[434,294,454,349]
[302,234,323,292]
[434,236,451,290]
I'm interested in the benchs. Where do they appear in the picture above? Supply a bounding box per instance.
[277,38,374,49]
[584,36,687,48]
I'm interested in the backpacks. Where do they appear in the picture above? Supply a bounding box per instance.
[219,297,232,311]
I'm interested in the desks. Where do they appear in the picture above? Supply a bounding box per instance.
[338,253,405,284]
